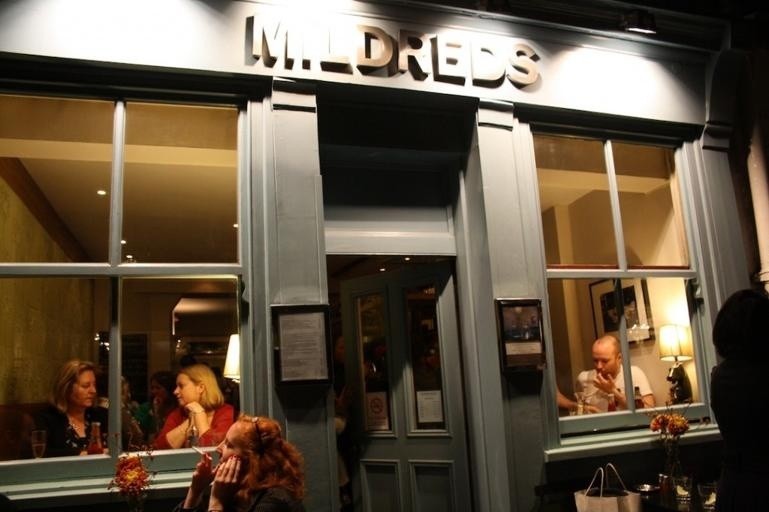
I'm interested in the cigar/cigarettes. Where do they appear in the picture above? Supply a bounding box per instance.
[191,444,213,461]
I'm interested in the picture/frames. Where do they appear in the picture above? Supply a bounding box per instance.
[588,274,655,349]
[269,302,333,388]
[494,297,549,373]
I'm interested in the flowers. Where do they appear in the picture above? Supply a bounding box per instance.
[105,426,160,512]
[640,392,690,474]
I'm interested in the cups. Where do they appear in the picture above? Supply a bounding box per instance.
[674,478,692,501]
[697,483,718,511]
[567,391,590,417]
[31,430,47,458]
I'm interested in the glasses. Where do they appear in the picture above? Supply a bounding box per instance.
[251,414,264,451]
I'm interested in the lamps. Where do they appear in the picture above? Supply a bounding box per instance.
[655,321,694,406]
[222,333,241,385]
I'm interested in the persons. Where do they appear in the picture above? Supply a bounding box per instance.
[176,411,307,511]
[149,363,234,450]
[132,368,173,439]
[48,356,103,458]
[96,374,146,441]
[554,382,603,416]
[332,356,375,512]
[707,285,768,511]
[570,334,656,416]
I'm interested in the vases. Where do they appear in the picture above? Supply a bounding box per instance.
[653,468,683,511]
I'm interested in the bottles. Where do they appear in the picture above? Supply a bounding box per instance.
[185,411,199,447]
[607,394,616,412]
[637,474,670,495]
[634,386,644,408]
[88,422,106,455]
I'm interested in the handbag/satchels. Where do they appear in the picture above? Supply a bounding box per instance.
[572,462,644,511]
